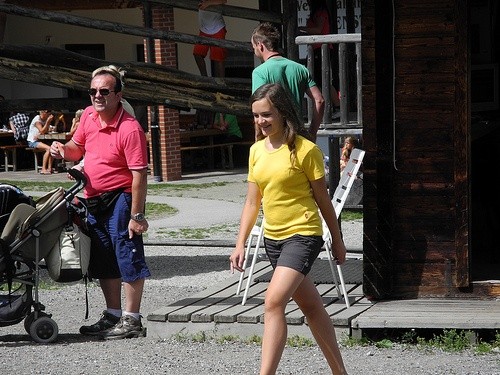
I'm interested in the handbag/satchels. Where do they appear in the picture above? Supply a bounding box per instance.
[44,218,91,283]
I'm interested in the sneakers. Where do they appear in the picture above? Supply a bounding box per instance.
[100,315,147,340]
[80,310,121,335]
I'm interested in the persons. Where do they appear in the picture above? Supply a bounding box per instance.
[9,112,30,167]
[50,110,66,170]
[192,0,227,78]
[229,84,349,375]
[68,65,136,187]
[70,109,84,135]
[214,112,243,168]
[27,109,53,174]
[251,21,325,145]
[340,135,357,173]
[50,68,151,340]
[306,0,340,120]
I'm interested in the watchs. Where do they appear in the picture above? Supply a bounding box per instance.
[130,213,145,221]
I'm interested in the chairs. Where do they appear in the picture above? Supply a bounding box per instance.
[236,148,366,308]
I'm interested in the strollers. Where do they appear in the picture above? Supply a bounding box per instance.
[0,168,86,343]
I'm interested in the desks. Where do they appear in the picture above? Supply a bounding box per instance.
[180,127,227,169]
[39,133,73,173]
[0,131,17,172]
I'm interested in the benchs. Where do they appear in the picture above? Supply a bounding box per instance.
[181,140,252,168]
[25,147,44,172]
[0,144,29,171]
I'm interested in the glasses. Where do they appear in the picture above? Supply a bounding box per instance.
[88,88,115,96]
